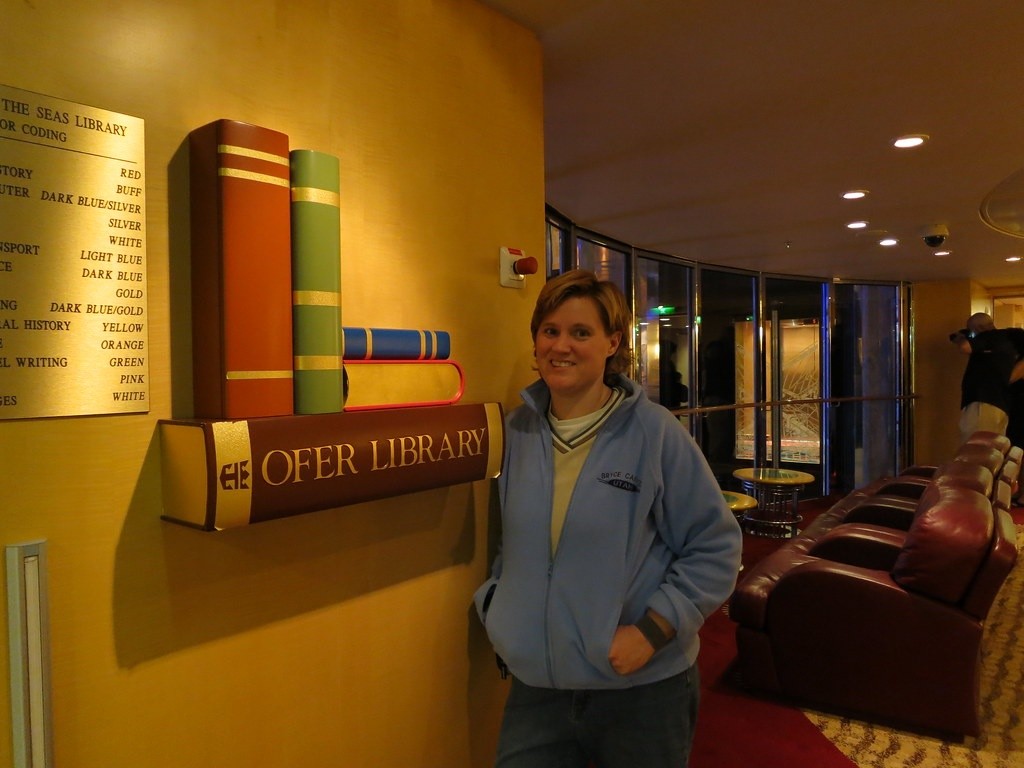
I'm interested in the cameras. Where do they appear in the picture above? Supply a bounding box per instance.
[949,328,975,342]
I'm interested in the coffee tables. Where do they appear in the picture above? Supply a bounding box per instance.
[721,490,758,522]
[733,468,815,539]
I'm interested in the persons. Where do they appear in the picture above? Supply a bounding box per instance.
[700,341,733,465]
[471,267,744,768]
[662,339,681,420]
[952,313,1024,510]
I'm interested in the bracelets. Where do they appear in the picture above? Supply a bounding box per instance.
[635,615,668,652]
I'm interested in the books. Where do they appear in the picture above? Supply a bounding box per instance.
[340,326,453,358]
[174,119,293,421]
[289,146,348,415]
[155,401,507,532]
[342,356,466,411]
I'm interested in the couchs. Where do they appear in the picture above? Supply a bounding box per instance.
[729,431,1024,745]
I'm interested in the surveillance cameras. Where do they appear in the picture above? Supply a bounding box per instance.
[919,226,951,247]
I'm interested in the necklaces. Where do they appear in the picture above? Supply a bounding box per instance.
[603,389,614,407]
